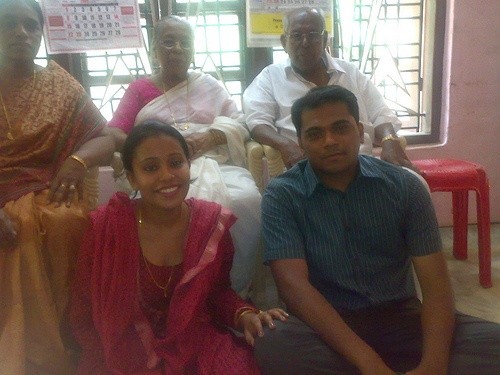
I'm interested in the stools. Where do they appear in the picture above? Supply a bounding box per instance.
[408,159,494,289]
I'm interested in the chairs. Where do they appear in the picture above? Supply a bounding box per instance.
[76,134,406,311]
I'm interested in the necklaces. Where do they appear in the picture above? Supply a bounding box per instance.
[158,73,191,131]
[0,67,38,141]
[138,201,187,299]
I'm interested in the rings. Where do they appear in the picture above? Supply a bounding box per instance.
[70,185,77,192]
[260,310,268,320]
[59,184,68,189]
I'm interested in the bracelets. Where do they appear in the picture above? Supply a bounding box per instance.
[209,129,222,148]
[238,308,257,323]
[70,154,88,171]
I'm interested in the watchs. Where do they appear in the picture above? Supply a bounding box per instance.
[381,134,400,144]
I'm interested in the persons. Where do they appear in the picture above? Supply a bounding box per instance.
[241,6,413,170]
[69,118,290,375]
[0,0,116,375]
[252,86,500,375]
[108,14,263,303]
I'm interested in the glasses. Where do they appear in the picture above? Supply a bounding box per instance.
[158,38,192,49]
[286,28,325,43]
